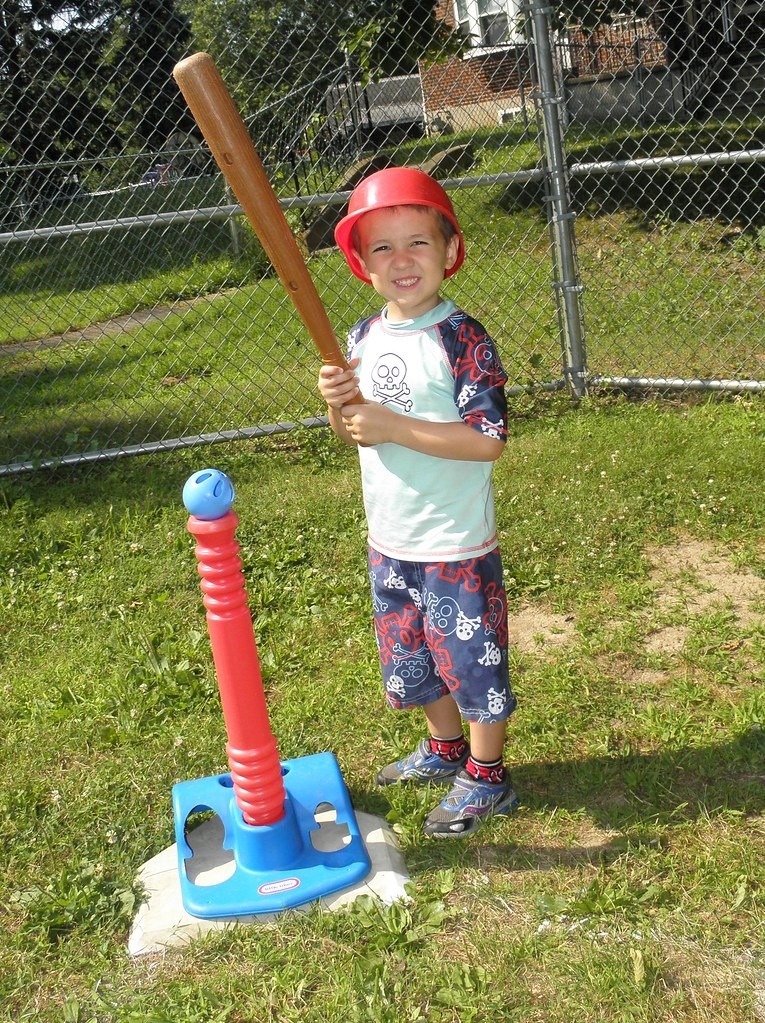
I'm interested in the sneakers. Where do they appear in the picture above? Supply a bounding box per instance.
[424,766,520,836]
[376,738,471,785]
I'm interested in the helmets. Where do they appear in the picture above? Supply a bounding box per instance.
[334,166,465,285]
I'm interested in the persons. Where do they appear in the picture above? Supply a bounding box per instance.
[318,166,517,839]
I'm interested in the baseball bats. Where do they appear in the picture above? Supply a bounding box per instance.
[168,48,383,450]
